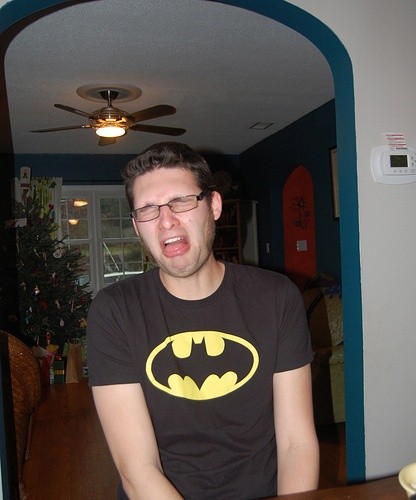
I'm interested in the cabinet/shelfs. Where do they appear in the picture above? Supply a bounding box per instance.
[210,198,250,265]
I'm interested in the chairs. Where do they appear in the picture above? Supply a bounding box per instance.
[0,329,42,500]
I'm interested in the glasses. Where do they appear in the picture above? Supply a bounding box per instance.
[130,191,205,222]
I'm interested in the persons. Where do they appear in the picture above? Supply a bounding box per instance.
[85,141,320,500]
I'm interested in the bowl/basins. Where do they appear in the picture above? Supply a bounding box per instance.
[398,462,416,500]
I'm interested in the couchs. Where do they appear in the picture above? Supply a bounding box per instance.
[300,272,345,438]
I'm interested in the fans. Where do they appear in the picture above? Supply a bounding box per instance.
[27,89,187,147]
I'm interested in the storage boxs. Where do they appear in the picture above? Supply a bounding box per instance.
[53,355,68,384]
